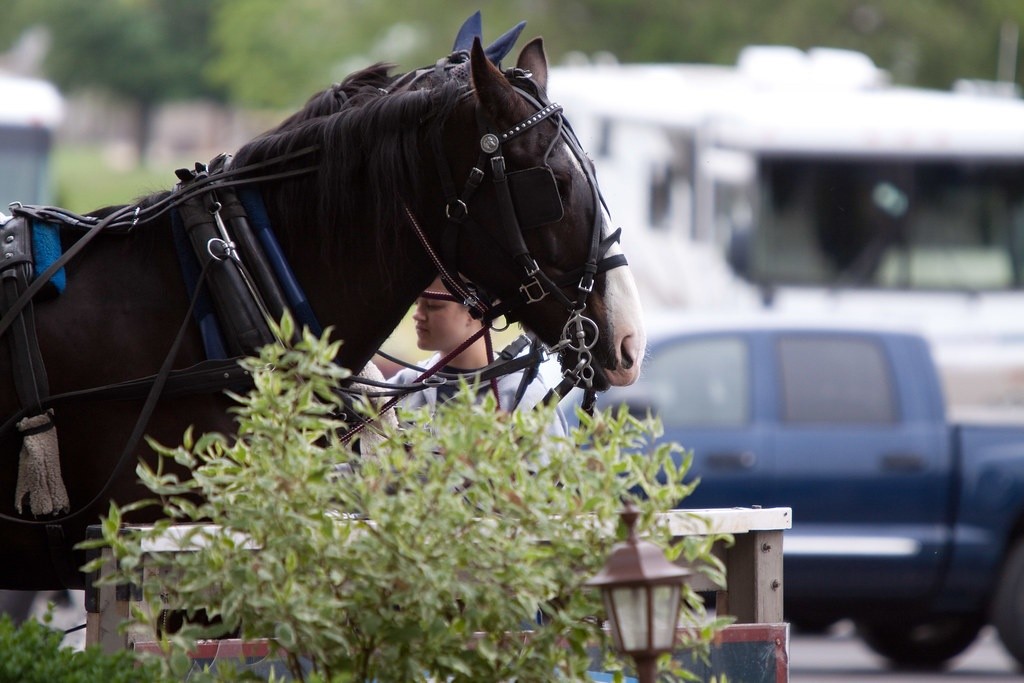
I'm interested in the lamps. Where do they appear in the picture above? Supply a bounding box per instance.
[581,494,693,657]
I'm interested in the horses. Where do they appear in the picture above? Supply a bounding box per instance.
[0,11,647,591]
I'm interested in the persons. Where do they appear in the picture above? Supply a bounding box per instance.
[388,269,576,683]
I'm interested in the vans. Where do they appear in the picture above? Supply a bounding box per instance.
[521,39,1024,667]
